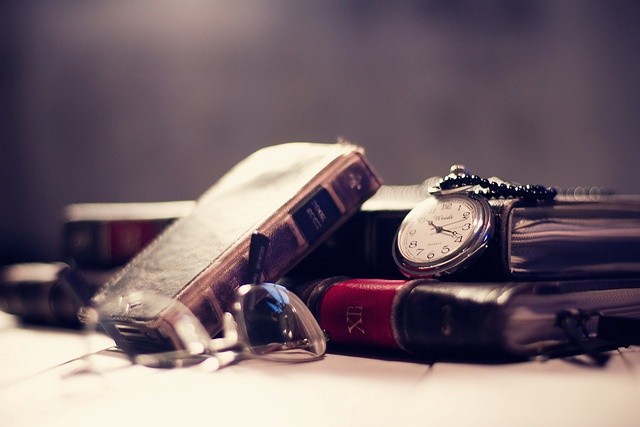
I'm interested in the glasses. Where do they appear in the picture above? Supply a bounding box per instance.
[85,231,326,377]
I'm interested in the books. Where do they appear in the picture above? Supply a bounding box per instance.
[0,261,640,364]
[58,184,640,280]
[77,142,385,354]
